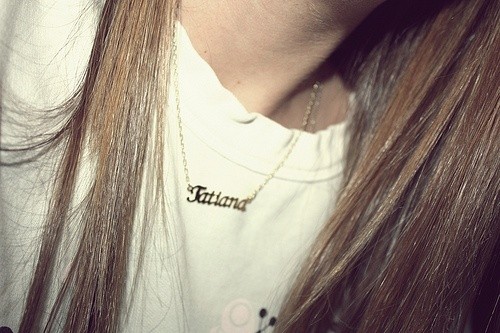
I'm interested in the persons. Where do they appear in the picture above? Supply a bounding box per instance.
[0,1,500,333]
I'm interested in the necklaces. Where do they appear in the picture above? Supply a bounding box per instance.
[170,0,330,212]
